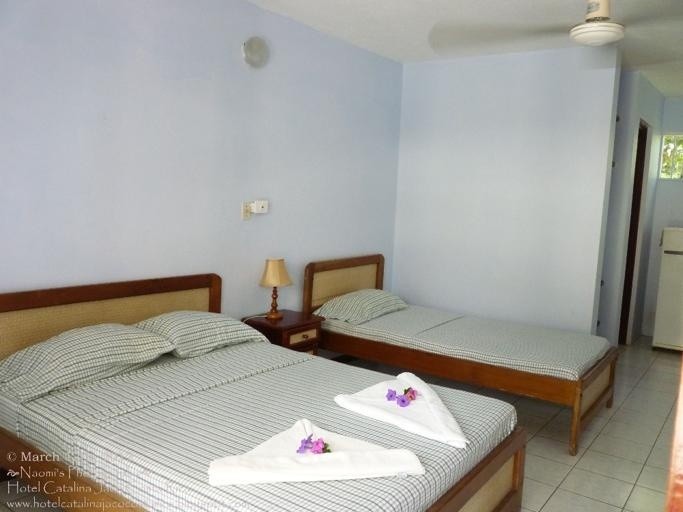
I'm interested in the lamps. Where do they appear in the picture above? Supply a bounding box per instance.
[260,257,293,321]
[569,2,627,49]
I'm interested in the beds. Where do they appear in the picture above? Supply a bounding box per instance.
[1,274,527,511]
[305,251,621,456]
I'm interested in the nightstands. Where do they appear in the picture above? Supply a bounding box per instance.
[244,308,326,351]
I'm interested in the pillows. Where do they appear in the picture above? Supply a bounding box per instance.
[309,288,410,327]
[3,311,275,400]
